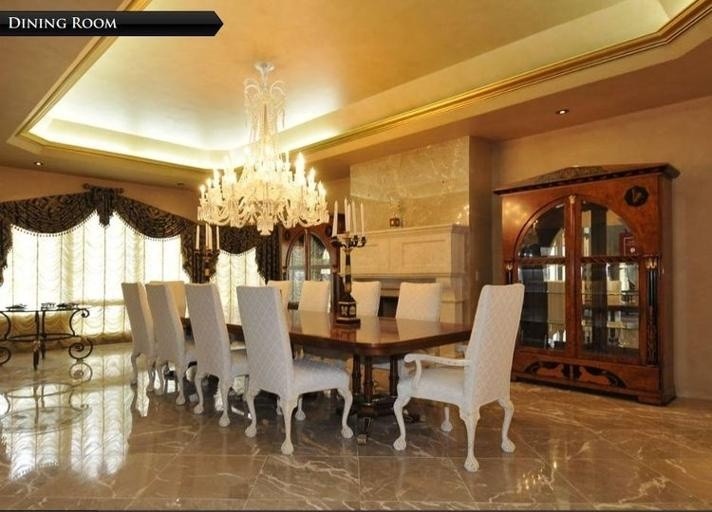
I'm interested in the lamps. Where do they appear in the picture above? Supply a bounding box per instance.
[193,59,328,237]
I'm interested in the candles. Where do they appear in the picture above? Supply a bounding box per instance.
[195,221,220,252]
[331,196,367,240]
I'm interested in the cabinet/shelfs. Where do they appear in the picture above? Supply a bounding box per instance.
[491,162,681,405]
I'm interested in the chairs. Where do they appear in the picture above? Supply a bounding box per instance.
[390,280,525,475]
[266,280,291,307]
[297,281,332,314]
[121,281,186,395]
[232,284,356,456]
[394,281,445,370]
[182,281,284,427]
[143,282,223,406]
[342,280,382,318]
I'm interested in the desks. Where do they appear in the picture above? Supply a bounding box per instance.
[0,306,94,365]
[170,303,475,448]
